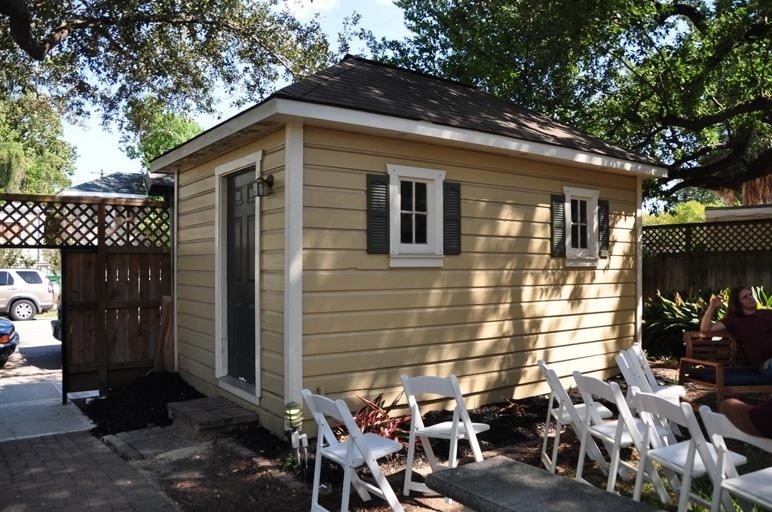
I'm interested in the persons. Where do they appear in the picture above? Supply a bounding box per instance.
[700,285,772,372]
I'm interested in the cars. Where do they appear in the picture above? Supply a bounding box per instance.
[0,269,54,321]
[0,318,20,368]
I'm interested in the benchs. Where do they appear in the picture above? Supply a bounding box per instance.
[425,453,660,511]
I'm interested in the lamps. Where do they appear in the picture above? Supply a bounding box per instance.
[251,171,274,196]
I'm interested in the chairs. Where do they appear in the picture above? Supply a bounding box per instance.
[615,340,693,490]
[298,373,491,512]
[570,368,674,504]
[536,359,630,483]
[677,330,770,422]
[626,382,748,512]
[698,403,771,511]
